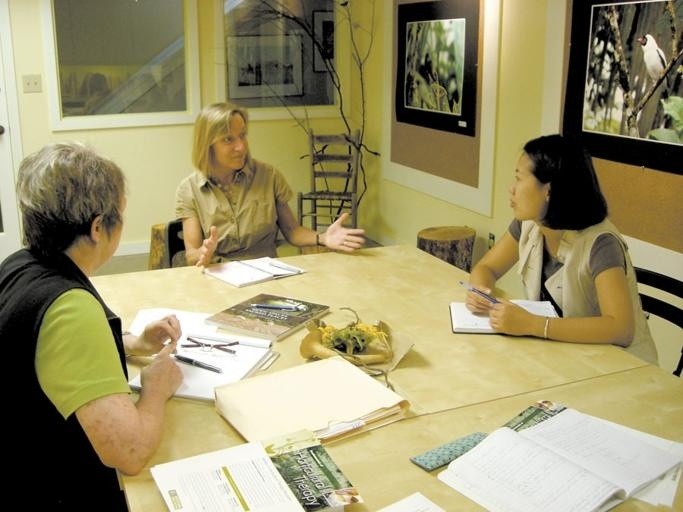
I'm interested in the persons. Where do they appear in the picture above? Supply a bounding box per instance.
[464,132,660,369]
[61,71,165,116]
[0,141,185,510]
[174,101,366,268]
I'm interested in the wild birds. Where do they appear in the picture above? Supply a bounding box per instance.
[636,34,672,104]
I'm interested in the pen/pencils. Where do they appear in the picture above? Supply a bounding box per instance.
[269,261,302,274]
[174,354,223,373]
[459,280,500,305]
[250,303,303,313]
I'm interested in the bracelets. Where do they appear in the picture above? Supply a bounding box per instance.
[120,330,134,359]
[315,232,326,247]
[543,316,550,339]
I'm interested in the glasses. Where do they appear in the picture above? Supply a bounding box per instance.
[181,338,239,357]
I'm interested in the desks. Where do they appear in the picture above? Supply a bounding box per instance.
[77,243,682,512]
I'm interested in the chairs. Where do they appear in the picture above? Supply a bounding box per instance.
[166,218,206,267]
[631,266,682,377]
[295,129,360,231]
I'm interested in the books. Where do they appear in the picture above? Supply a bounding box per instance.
[449,299,560,333]
[437,398,683,512]
[197,254,307,289]
[202,293,330,343]
[131,337,274,403]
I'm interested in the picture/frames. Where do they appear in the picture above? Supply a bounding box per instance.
[394,0,480,139]
[562,0,683,176]
[224,9,334,100]
